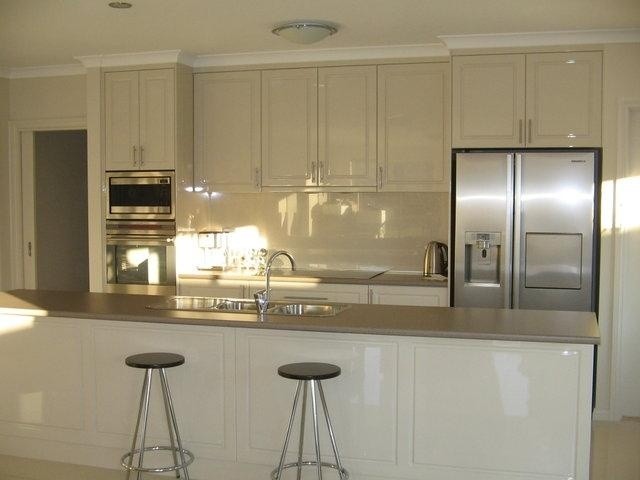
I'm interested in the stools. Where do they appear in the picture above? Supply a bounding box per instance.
[271,363,350,480]
[120,353,195,480]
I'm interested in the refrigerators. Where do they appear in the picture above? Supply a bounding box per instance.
[450,148,599,311]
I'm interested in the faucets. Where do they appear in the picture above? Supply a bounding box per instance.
[254,251,297,313]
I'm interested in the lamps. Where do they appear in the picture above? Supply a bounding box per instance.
[272,21,337,43]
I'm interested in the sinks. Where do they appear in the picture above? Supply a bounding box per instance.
[218,297,279,315]
[156,294,224,310]
[268,301,349,317]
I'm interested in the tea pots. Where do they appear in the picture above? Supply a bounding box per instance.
[423,241,448,277]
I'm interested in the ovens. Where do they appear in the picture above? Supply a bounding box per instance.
[106,171,175,220]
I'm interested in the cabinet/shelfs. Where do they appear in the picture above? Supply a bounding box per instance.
[261,65,377,192]
[377,63,450,193]
[194,70,260,194]
[178,278,448,308]
[451,52,602,148]
[104,62,192,171]
